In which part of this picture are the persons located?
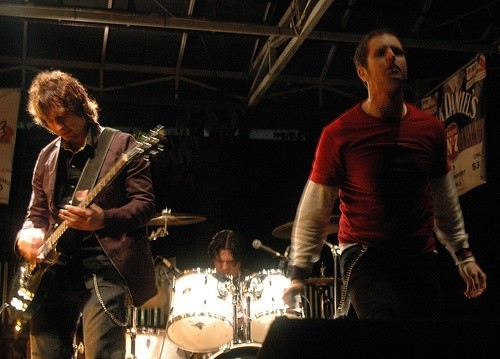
[207,229,250,277]
[282,29,487,321]
[13,71,158,359]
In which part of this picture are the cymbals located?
[303,277,337,289]
[143,213,207,226]
[271,215,341,240]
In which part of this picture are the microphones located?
[252,239,285,258]
[160,256,180,277]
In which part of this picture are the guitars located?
[5,123,169,324]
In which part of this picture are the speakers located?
[258,316,500,359]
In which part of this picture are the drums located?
[230,274,251,344]
[123,326,188,359]
[165,266,236,354]
[242,268,304,344]
[203,341,263,359]
